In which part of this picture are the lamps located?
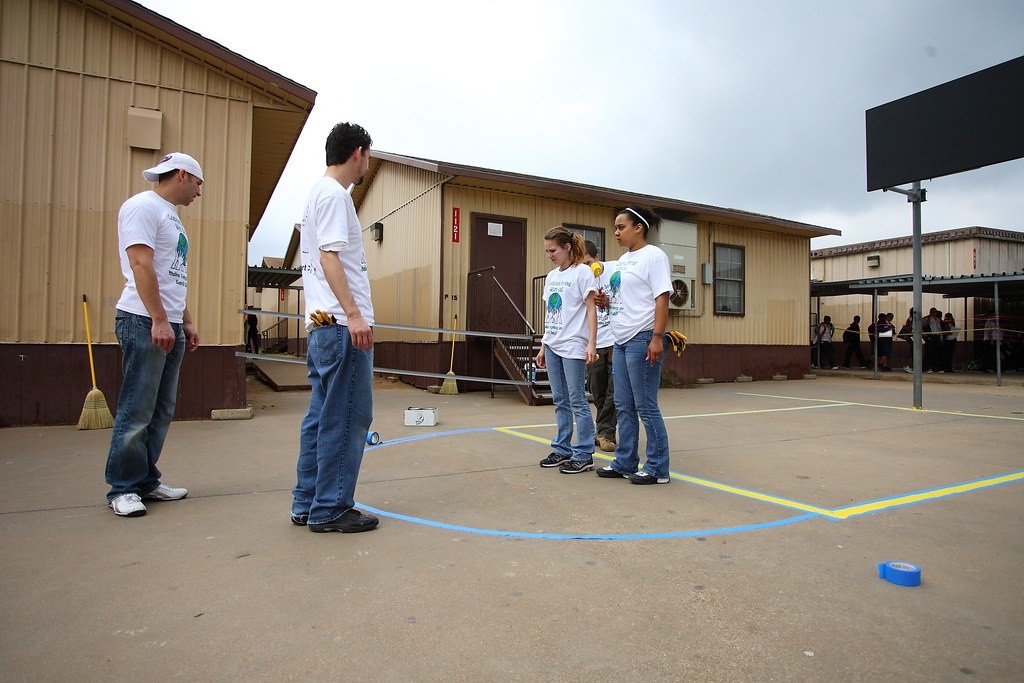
[866,254,880,267]
[370,222,383,241]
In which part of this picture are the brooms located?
[76,293,114,431]
[438,314,459,394]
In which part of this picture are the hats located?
[142,152,204,183]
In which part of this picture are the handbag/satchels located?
[897,317,913,341]
[814,323,825,337]
[946,324,961,340]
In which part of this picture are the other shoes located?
[811,363,821,369]
[884,366,892,372]
[860,365,869,369]
[986,370,993,374]
[936,369,944,374]
[1005,369,1016,372]
[830,365,838,370]
[843,365,851,370]
[925,368,933,374]
[903,365,914,374]
[878,363,884,372]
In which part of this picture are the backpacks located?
[868,322,885,339]
[843,326,859,343]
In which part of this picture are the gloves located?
[309,310,333,328]
[664,331,688,358]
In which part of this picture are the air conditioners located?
[668,274,696,309]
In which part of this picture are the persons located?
[582,240,619,452]
[842,315,868,369]
[903,307,957,374]
[594,205,673,484]
[981,312,1016,373]
[103,151,206,518]
[290,125,381,533]
[535,225,597,475]
[869,312,895,372]
[811,315,840,369]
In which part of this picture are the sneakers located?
[142,482,189,501]
[540,453,572,468]
[109,493,147,517]
[309,509,379,533]
[595,464,631,479]
[596,432,616,452]
[629,470,670,484]
[290,514,308,525]
[559,456,594,474]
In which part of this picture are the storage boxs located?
[403,408,437,428]
